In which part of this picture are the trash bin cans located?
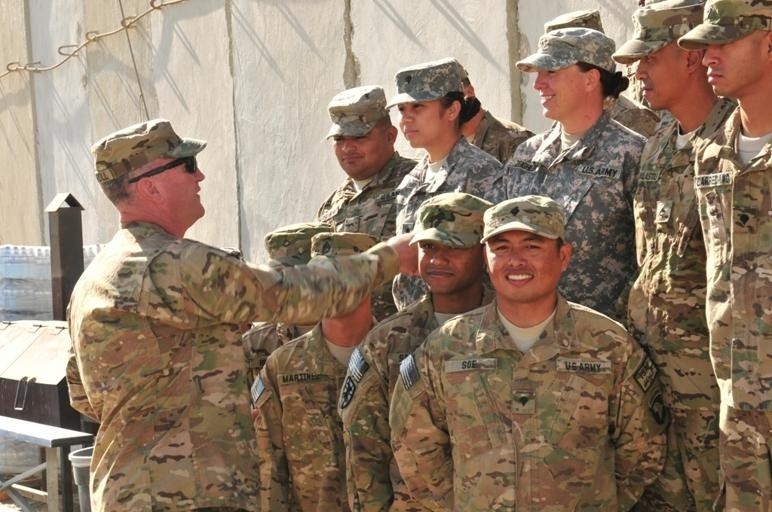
[67,446,94,512]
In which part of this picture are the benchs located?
[0,414,95,511]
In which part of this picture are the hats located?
[263,222,381,267]
[544,8,604,38]
[479,195,566,246]
[384,57,467,111]
[677,1,772,52]
[610,1,706,64]
[91,118,209,184]
[515,27,616,74]
[408,192,495,249]
[326,85,388,141]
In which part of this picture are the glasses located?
[127,156,198,183]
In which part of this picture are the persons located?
[453,57,536,164]
[497,28,650,330]
[545,10,660,139]
[64,119,418,510]
[611,2,726,509]
[338,193,495,510]
[384,57,503,312]
[241,223,334,389]
[250,231,380,510]
[313,85,419,319]
[676,2,771,510]
[390,197,666,510]
